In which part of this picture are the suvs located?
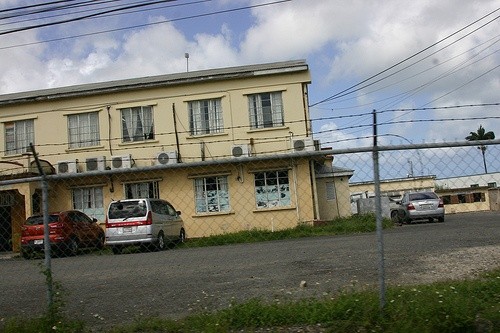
[19,209,105,259]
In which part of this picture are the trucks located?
[350,191,400,216]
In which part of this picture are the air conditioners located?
[84,155,107,172]
[110,154,132,169]
[57,158,80,175]
[313,139,321,151]
[230,143,251,157]
[291,138,315,152]
[154,150,178,166]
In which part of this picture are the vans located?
[104,199,186,255]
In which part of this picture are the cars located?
[396,190,445,223]
